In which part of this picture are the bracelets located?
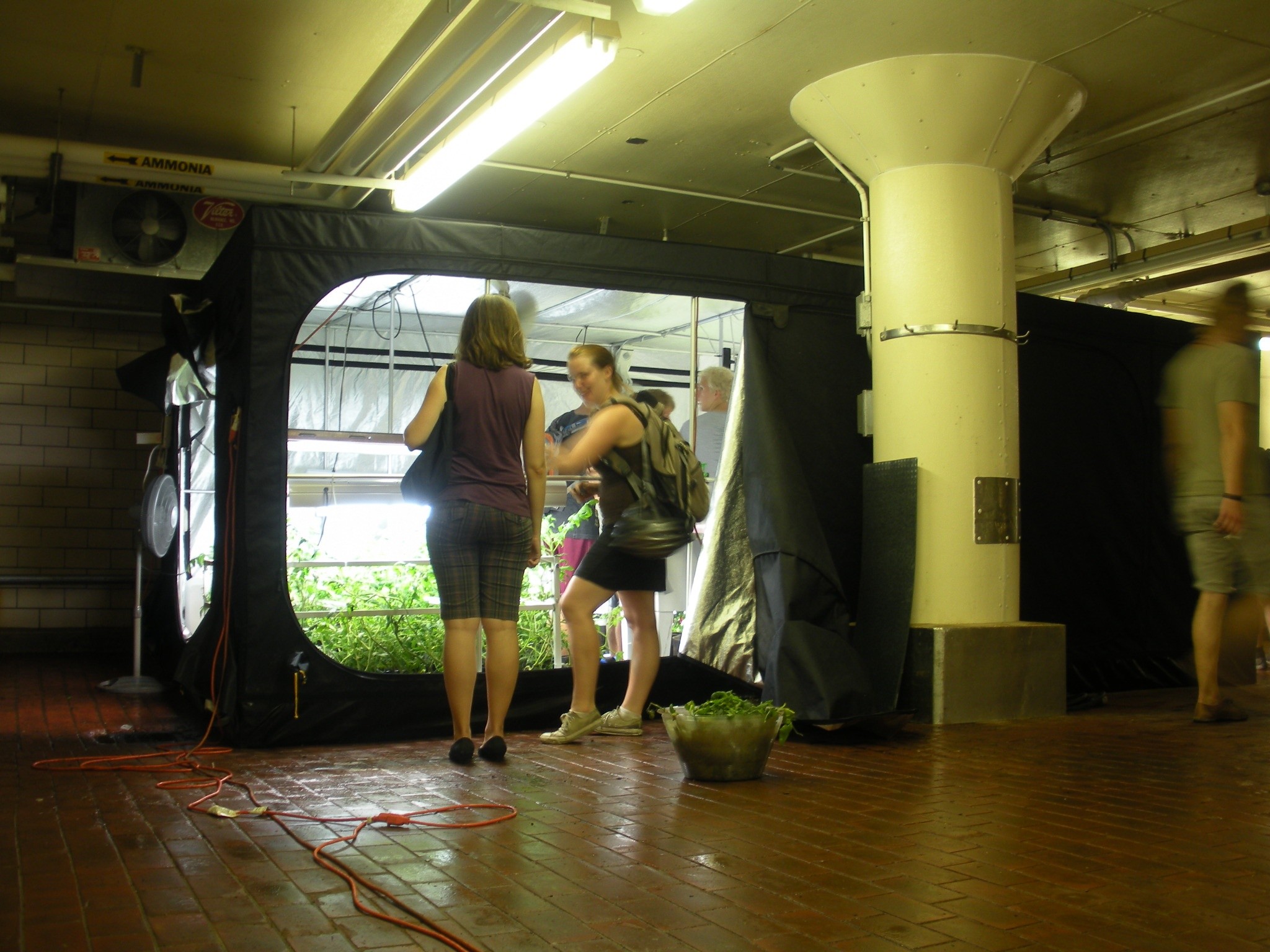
[1221,491,1243,503]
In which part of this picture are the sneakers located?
[540,708,601,744]
[594,705,642,736]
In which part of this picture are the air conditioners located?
[70,178,252,286]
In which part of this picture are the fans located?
[99,471,184,698]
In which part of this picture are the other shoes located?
[478,735,508,761]
[448,737,475,761]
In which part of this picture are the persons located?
[537,344,699,745]
[604,387,677,661]
[396,291,548,765]
[1160,279,1270,721]
[540,391,608,667]
[675,367,737,508]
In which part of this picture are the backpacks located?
[588,398,711,524]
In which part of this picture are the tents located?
[114,200,1263,754]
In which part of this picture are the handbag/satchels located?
[400,400,456,503]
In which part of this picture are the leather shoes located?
[1193,699,1248,723]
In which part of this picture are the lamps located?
[630,0,699,17]
[385,14,622,216]
